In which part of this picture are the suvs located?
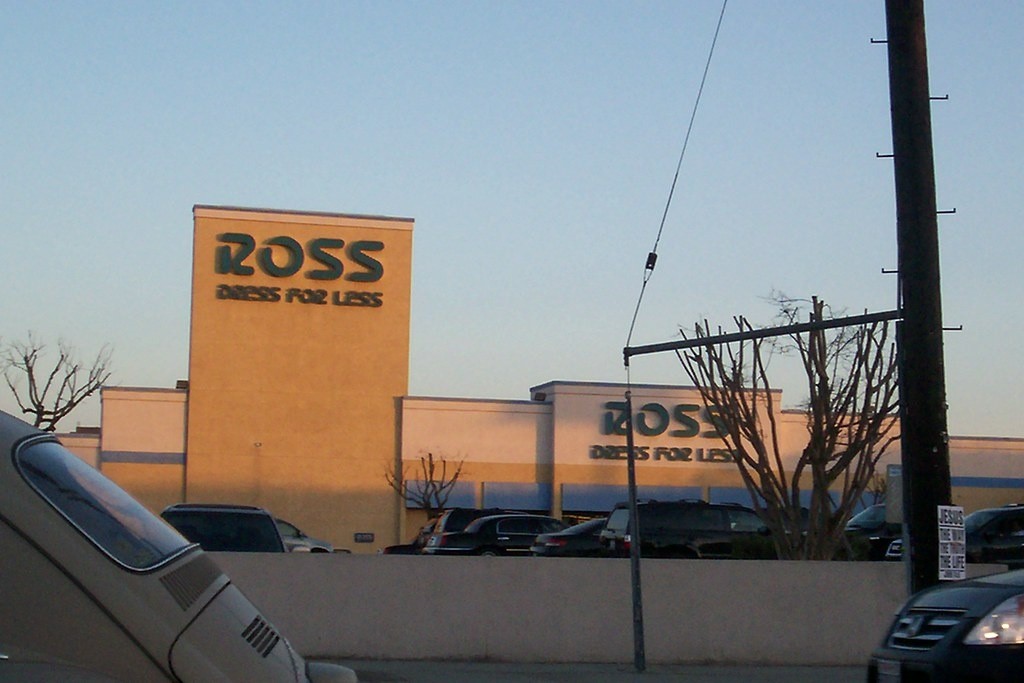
[160,504,351,555]
[381,500,834,561]
[827,500,1024,565]
[0,408,362,683]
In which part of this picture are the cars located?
[868,567,1024,683]
